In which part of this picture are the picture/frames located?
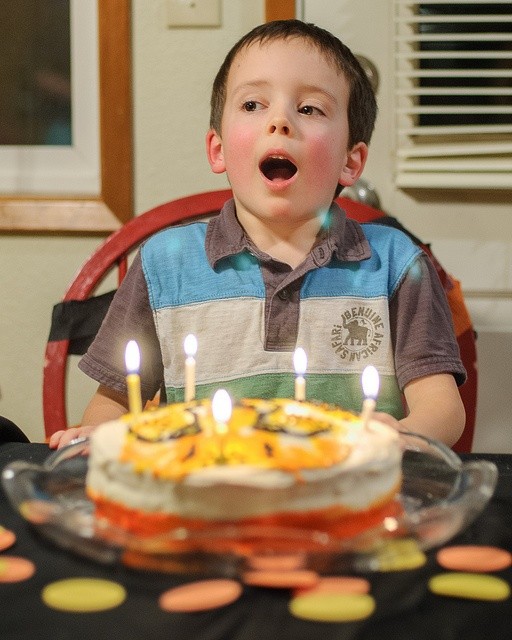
[0,0,135,237]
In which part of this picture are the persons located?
[49,22,467,452]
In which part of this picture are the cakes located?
[87,397,404,564]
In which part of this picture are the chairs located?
[42,187,477,457]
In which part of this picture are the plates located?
[3,429,497,585]
